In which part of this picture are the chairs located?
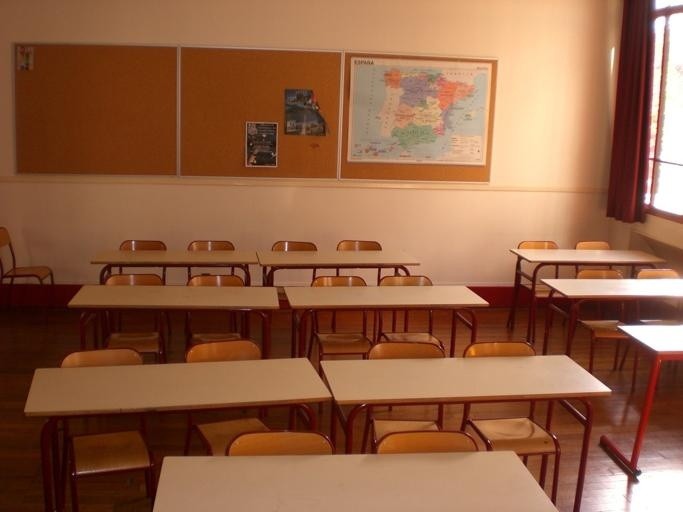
[0,227,58,323]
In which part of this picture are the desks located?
[23,357,333,512]
